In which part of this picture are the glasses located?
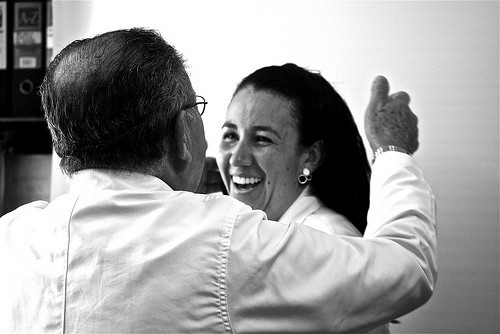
[182,95,208,117]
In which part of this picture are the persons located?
[215,63,390,334]
[0,28,440,334]
